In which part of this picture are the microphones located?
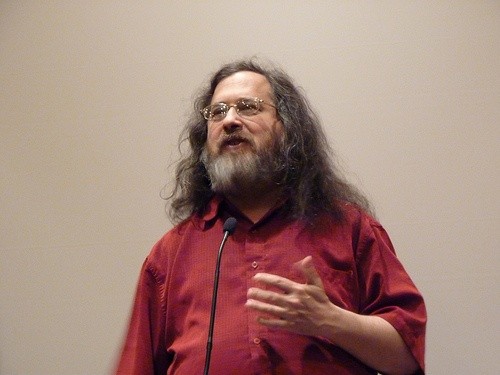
[203,217,237,375]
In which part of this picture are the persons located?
[114,60,428,375]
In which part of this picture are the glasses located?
[200,97,279,121]
